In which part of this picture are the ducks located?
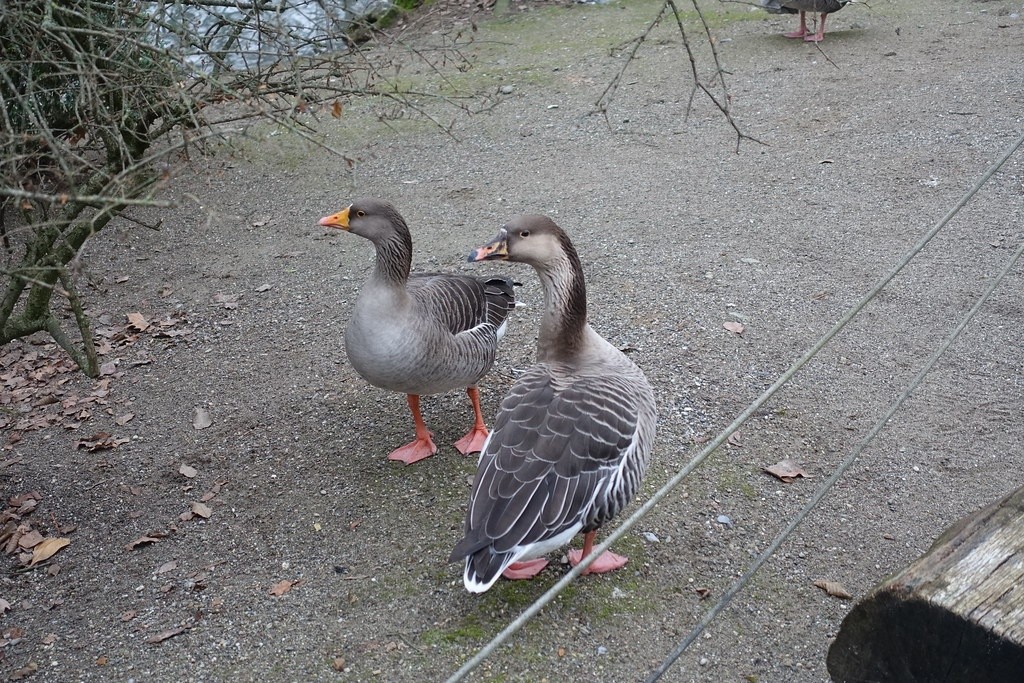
[446,211,657,593]
[760,0,852,43]
[316,196,523,467]
